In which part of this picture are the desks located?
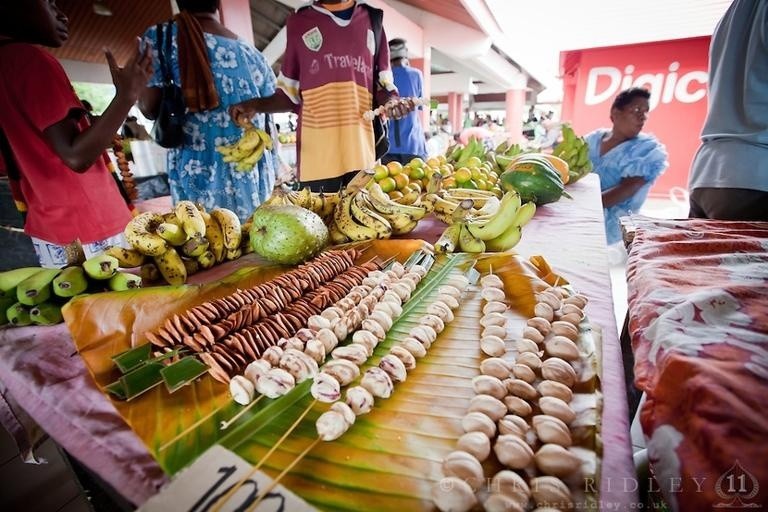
[1,169,639,512]
[616,210,767,511]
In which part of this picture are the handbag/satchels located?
[148,84,187,149]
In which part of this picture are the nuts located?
[145,248,589,512]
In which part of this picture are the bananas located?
[0,125,594,329]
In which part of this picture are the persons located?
[421,103,553,140]
[138,0,277,226]
[0,0,155,269]
[230,0,411,194]
[688,0,767,221]
[82,98,152,140]
[380,39,427,166]
[275,111,300,147]
[586,87,669,267]
